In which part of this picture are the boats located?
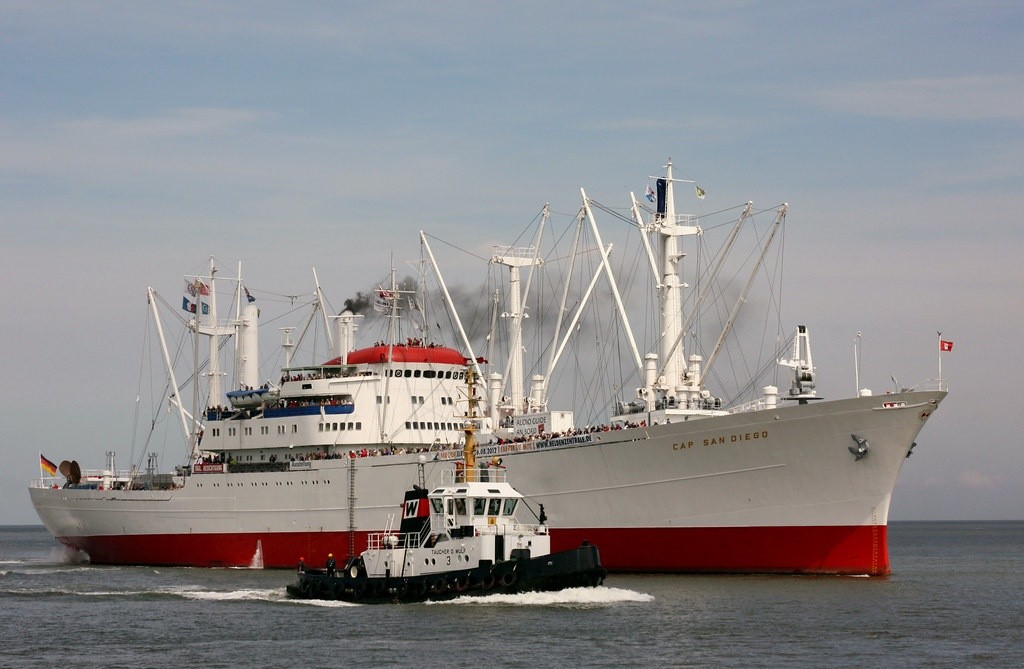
[226,389,281,408]
[286,360,611,606]
[25,164,947,582]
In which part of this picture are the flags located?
[182,277,210,316]
[40,453,58,476]
[941,341,953,352]
[646,184,658,202]
[695,185,708,199]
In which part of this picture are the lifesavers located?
[500,570,519,588]
[373,581,385,594]
[414,581,428,596]
[480,572,496,590]
[398,582,410,596]
[356,584,366,595]
[456,574,470,591]
[396,370,401,376]
[433,578,448,595]
[453,372,457,379]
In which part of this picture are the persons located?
[374,337,442,348]
[488,418,671,446]
[203,440,465,463]
[50,483,183,490]
[206,371,368,414]
